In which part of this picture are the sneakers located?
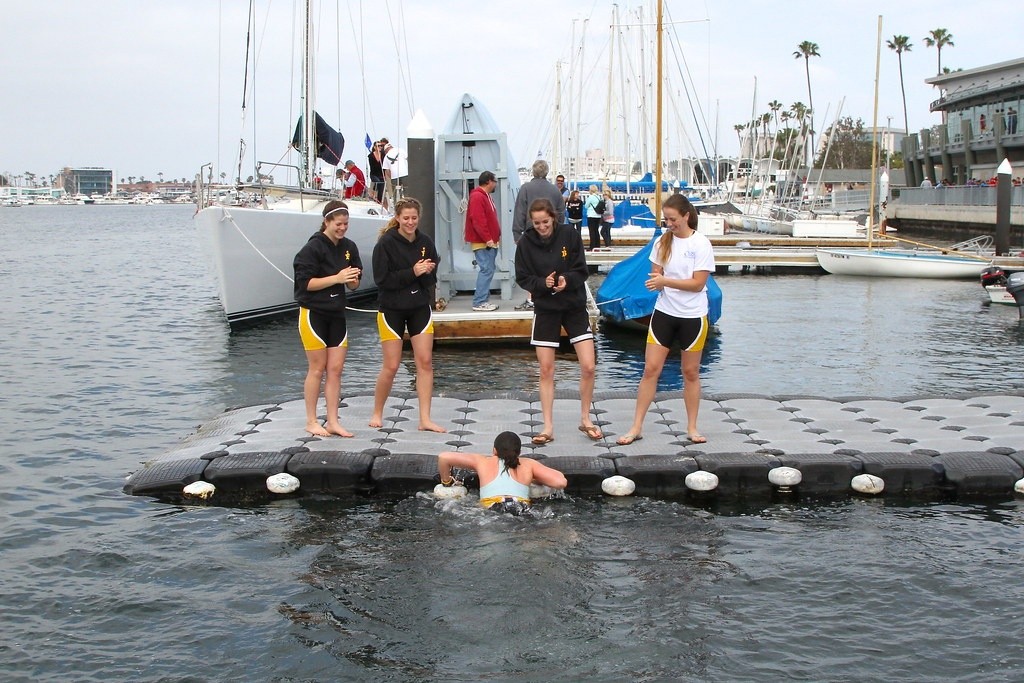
[473,302,499,310]
[515,299,535,311]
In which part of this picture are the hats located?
[336,169,343,179]
[344,160,354,169]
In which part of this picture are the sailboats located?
[190,0,422,329]
[597,0,724,333]
[522,2,864,237]
[816,15,995,278]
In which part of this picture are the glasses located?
[557,179,564,182]
[376,145,383,148]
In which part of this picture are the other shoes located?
[586,248,599,251]
[602,247,612,250]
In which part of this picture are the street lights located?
[886,116,895,174]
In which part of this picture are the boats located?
[980,268,1023,304]
[0,190,269,206]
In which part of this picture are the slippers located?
[578,426,603,438]
[616,435,642,445]
[531,434,555,444]
[685,435,706,443]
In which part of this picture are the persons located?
[336,160,365,200]
[437,432,567,512]
[513,160,565,311]
[567,191,582,235]
[616,195,717,444]
[368,198,446,432]
[980,115,989,139]
[515,199,604,444]
[967,176,1024,188]
[368,138,409,207]
[996,107,1017,134]
[293,201,362,437]
[585,184,600,252]
[920,176,952,189]
[464,171,502,311]
[600,190,615,247]
[555,176,569,224]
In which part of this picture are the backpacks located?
[590,195,606,214]
